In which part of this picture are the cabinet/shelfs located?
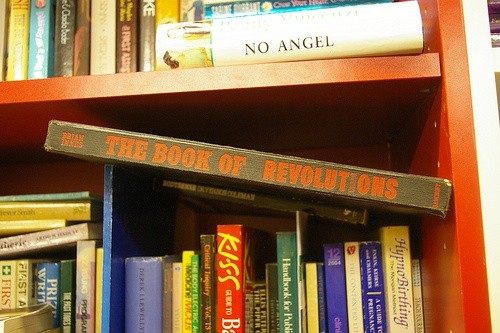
[465,0,500,333]
[0,0,493,333]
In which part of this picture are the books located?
[43,119,452,217]
[161,181,368,226]
[0,191,426,333]
[0,0,425,82]
